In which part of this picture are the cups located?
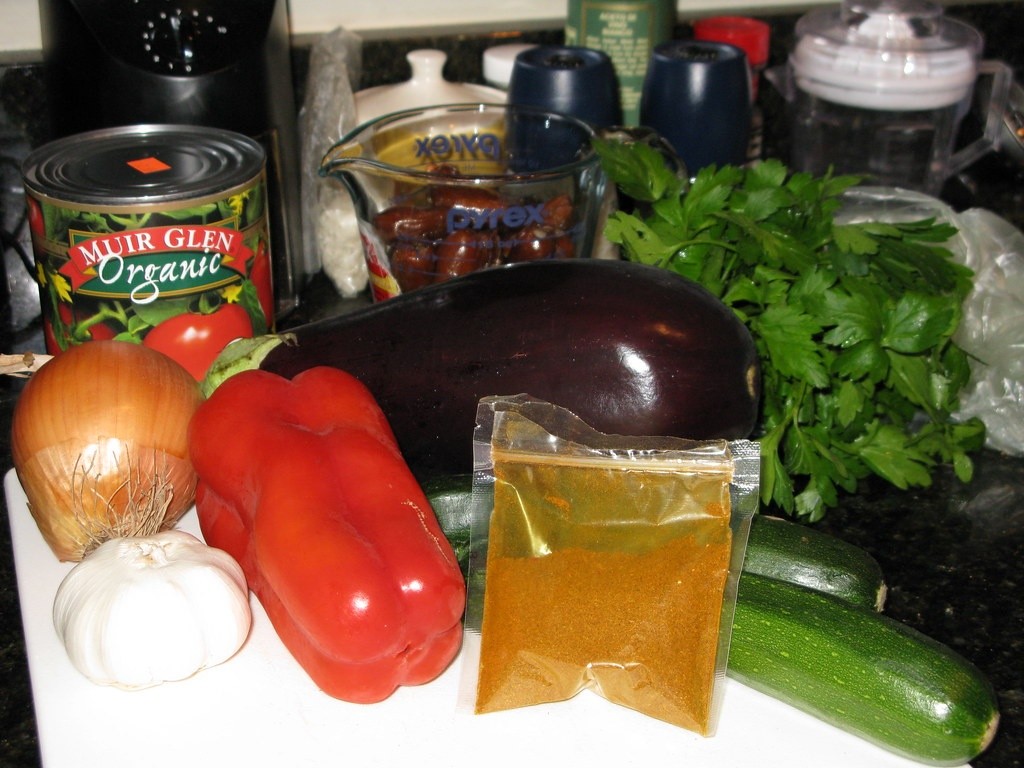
[353,49,507,129]
[640,40,753,179]
[504,46,624,132]
[110,56,241,132]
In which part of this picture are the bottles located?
[482,43,537,92]
[694,16,771,168]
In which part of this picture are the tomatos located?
[46,243,268,385]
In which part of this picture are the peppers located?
[185,366,469,703]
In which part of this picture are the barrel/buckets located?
[19,120,278,360]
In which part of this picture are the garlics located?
[52,529,252,689]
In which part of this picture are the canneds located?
[20,123,270,382]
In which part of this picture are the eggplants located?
[199,255,765,483]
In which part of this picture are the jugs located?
[319,102,688,304]
[764,0,1012,199]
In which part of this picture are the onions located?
[10,340,198,563]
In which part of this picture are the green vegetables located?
[591,131,987,519]
